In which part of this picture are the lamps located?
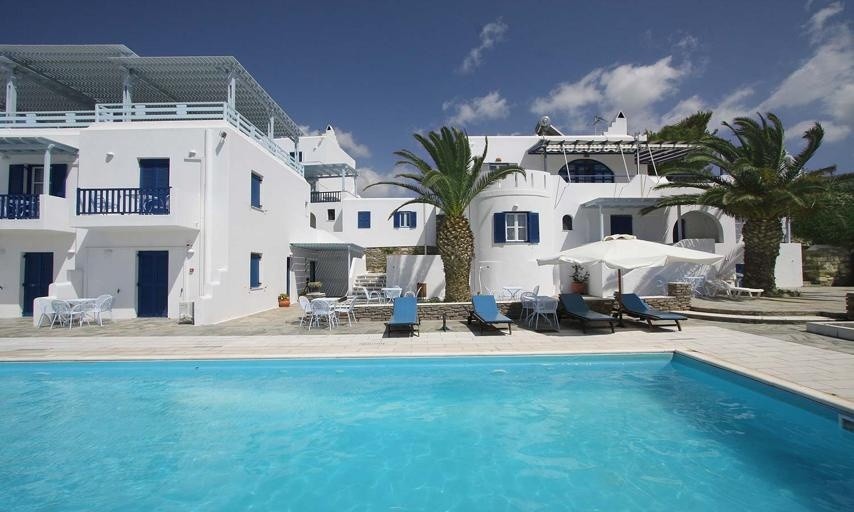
[219,130,227,143]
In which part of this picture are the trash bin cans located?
[417,282,427,303]
[736,264,745,289]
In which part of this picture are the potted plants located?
[277,293,290,307]
[307,281,321,292]
[567,264,590,294]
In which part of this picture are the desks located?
[380,286,402,304]
[305,292,325,298]
[309,297,341,328]
[59,298,99,327]
[525,296,554,330]
[502,287,523,301]
[128,193,158,215]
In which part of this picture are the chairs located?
[335,295,357,327]
[468,294,513,335]
[306,299,338,331]
[534,295,561,331]
[614,293,688,331]
[298,295,319,328]
[362,287,381,304]
[36,298,56,328]
[382,295,420,340]
[705,278,765,298]
[85,295,113,326]
[50,299,83,330]
[557,293,618,334]
[519,285,539,322]
[89,192,116,212]
[403,286,422,297]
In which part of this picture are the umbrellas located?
[535,234,723,327]
